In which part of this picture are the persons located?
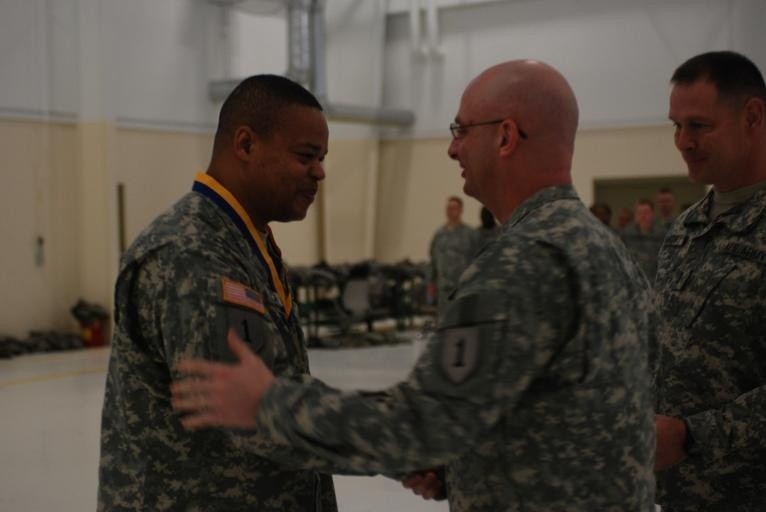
[426,194,473,327]
[94,72,342,512]
[469,203,501,252]
[163,57,661,511]
[588,186,696,289]
[641,44,766,512]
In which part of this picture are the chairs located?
[285,258,437,348]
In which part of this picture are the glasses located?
[450,119,528,141]
